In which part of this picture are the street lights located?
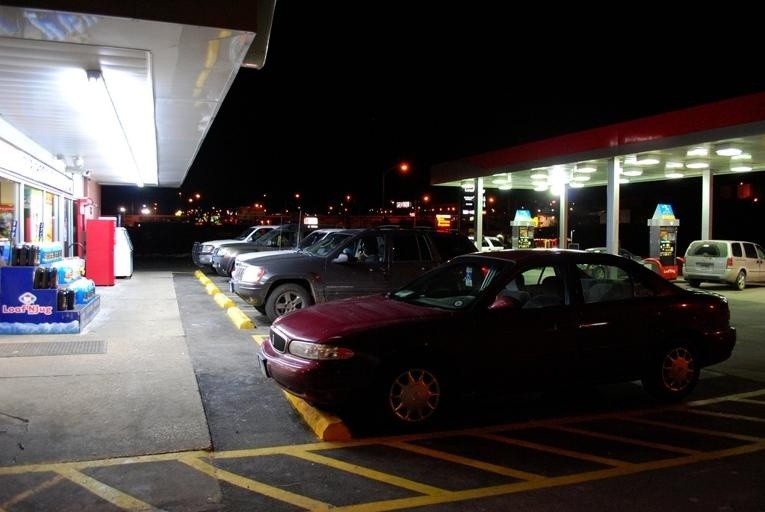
[295,192,300,213]
[413,191,431,227]
[187,191,201,224]
[345,196,351,217]
[485,196,495,234]
[381,162,412,227]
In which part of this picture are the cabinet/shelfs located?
[0,262,102,336]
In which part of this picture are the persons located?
[330,238,353,255]
[276,233,288,241]
[255,230,265,239]
[363,246,379,263]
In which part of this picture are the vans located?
[680,239,765,291]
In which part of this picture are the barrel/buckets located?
[11,241,96,312]
